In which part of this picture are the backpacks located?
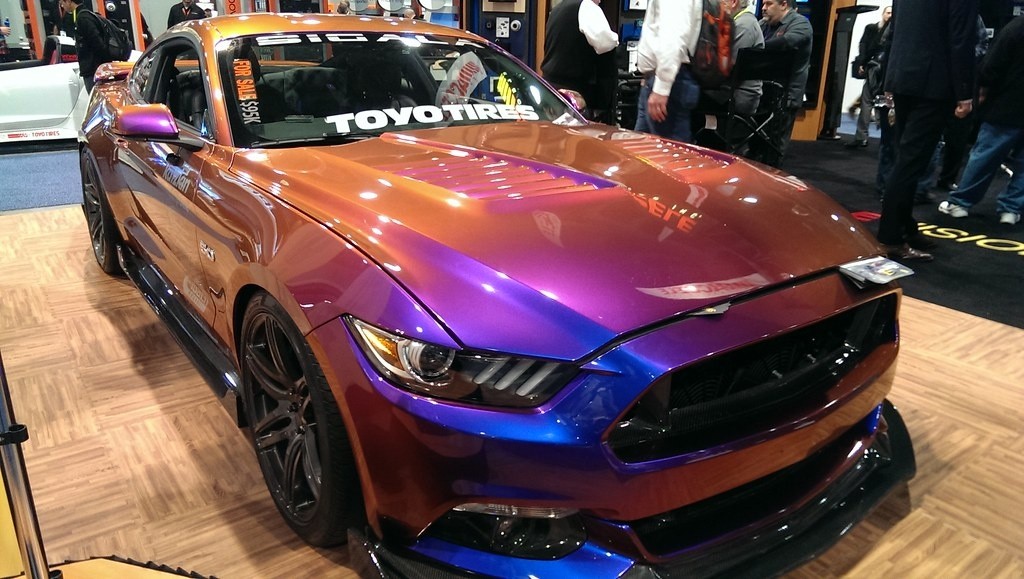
[76,9,132,61]
[688,0,737,90]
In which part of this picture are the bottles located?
[874,94,894,108]
[3,17,10,38]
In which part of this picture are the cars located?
[75,13,920,578]
[0,36,146,143]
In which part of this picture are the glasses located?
[60,0,64,7]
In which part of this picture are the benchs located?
[169,67,346,126]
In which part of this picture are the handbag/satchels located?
[852,60,868,79]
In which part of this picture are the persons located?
[60,0,113,96]
[331,0,357,55]
[848,0,1024,265]
[0,15,11,63]
[632,0,814,171]
[540,0,620,124]
[167,0,218,60]
[403,9,418,19]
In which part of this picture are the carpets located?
[0,152,84,210]
[779,134,1024,330]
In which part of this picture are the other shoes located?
[938,179,959,191]
[912,189,936,201]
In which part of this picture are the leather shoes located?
[846,139,868,146]
[904,229,937,249]
[880,242,934,263]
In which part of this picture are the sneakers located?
[939,200,968,218]
[1000,212,1021,224]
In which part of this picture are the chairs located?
[347,43,417,113]
[192,43,293,130]
[693,47,798,164]
